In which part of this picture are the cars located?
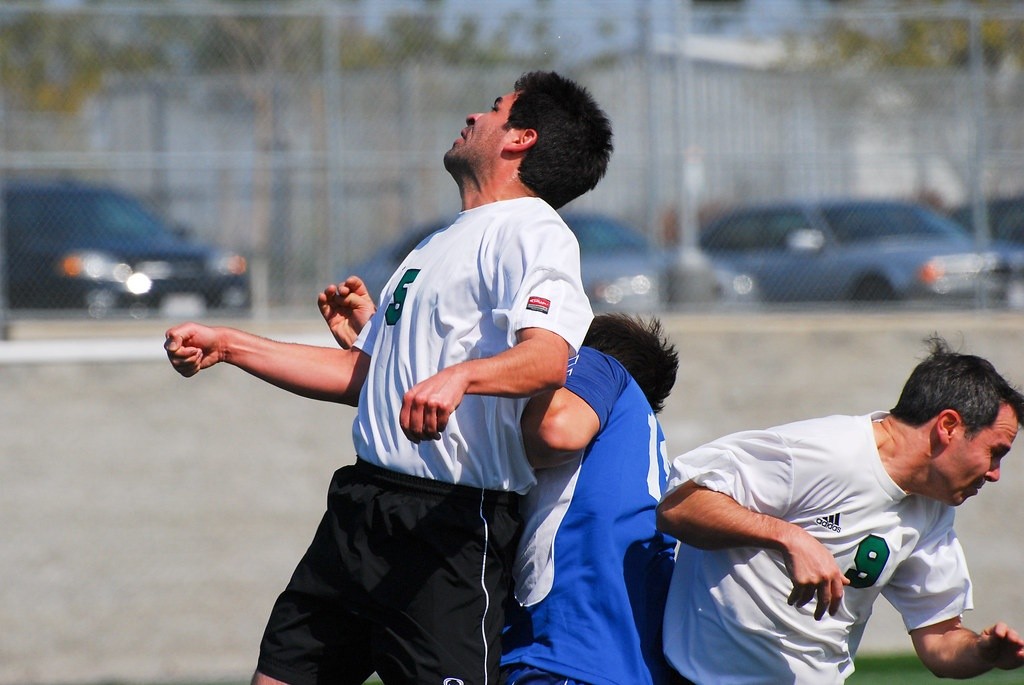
[331,205,754,311]
[1,179,247,313]
[690,197,1024,305]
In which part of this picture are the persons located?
[165,70,613,685]
[313,274,678,685]
[655,334,1023,684]
